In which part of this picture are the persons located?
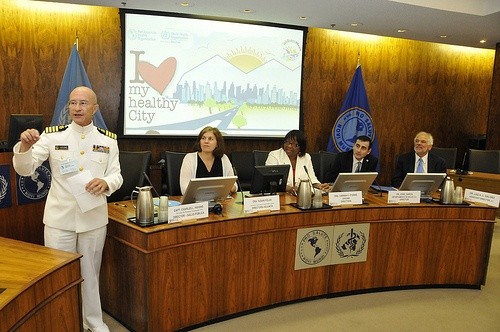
[12,85,123,332]
[180,126,239,195]
[394,132,446,189]
[264,130,331,194]
[336,136,378,185]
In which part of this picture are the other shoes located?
[84,324,110,332]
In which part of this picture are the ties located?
[416,159,423,173]
[356,162,360,171]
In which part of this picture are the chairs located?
[461,148,500,174]
[432,147,457,169]
[104,150,339,203]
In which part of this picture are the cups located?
[313,191,322,208]
[454,187,463,204]
[158,197,168,221]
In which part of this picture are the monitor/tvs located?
[7,114,44,145]
[249,165,290,195]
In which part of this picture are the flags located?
[49,44,107,130]
[328,64,380,172]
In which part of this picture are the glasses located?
[69,100,96,108]
[284,141,298,147]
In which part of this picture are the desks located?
[99,191,499,332]
[0,237,85,332]
[447,169,500,219]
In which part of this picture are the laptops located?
[180,176,238,208]
[331,172,378,201]
[399,173,446,199]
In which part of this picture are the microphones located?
[304,166,315,193]
[456,149,468,174]
[233,167,244,205]
[143,171,160,199]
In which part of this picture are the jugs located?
[440,177,454,204]
[292,180,312,209]
[131,186,154,224]
